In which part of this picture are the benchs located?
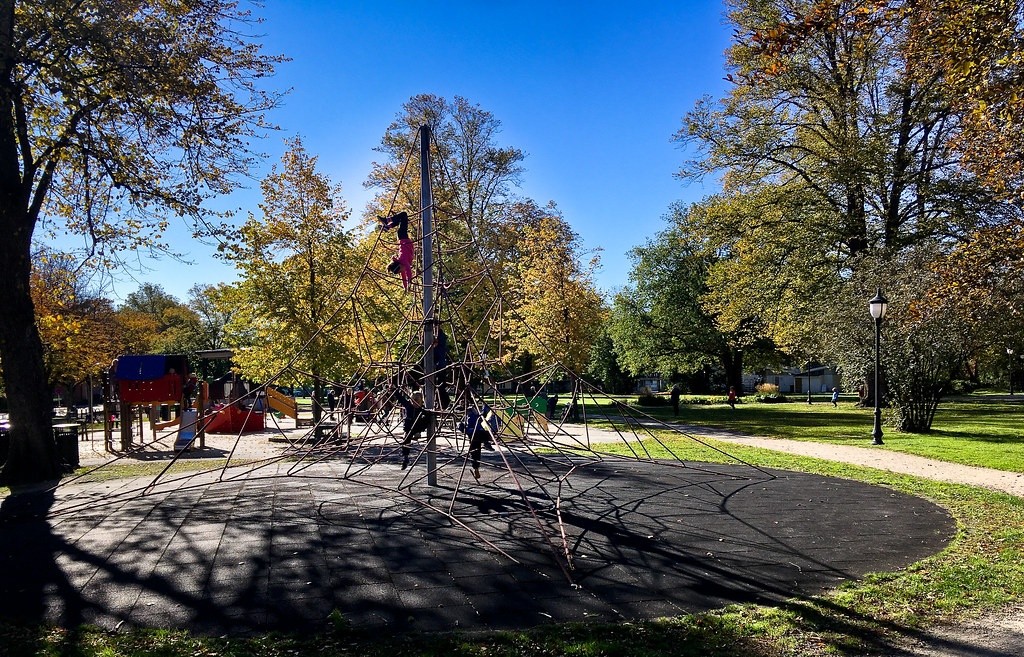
[316,422,337,440]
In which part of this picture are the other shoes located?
[377,216,389,226]
[413,432,421,440]
[474,471,480,478]
[330,418,337,421]
[386,424,392,426]
[401,459,412,470]
[484,445,495,451]
[377,421,381,425]
[377,224,389,232]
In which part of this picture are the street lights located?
[478,349,487,398]
[1007,348,1014,395]
[806,347,813,404]
[868,288,889,445]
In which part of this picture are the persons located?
[831,387,838,408]
[430,326,453,410]
[727,386,735,412]
[389,383,429,470]
[273,385,292,396]
[342,378,413,426]
[465,403,495,480]
[327,390,337,421]
[547,394,559,420]
[377,211,415,295]
[670,384,679,417]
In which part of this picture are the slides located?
[154,394,200,453]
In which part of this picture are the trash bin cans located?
[52,424,81,465]
